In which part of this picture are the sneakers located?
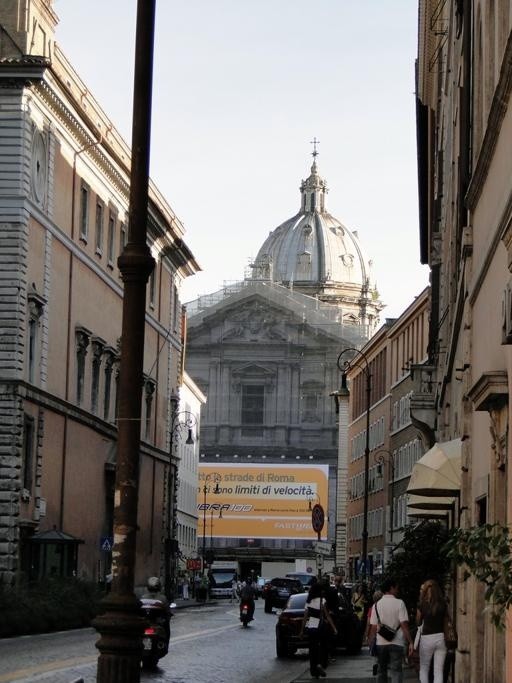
[373,664,378,675]
[312,665,326,679]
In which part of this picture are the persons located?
[298,583,339,678]
[316,575,383,647]
[140,576,171,610]
[237,575,258,597]
[178,570,217,601]
[238,577,257,620]
[415,579,450,682]
[229,575,240,603]
[367,578,414,682]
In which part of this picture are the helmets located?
[246,577,252,584]
[146,577,161,592]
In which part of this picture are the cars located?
[286,572,317,593]
[263,577,306,613]
[274,592,363,659]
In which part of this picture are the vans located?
[207,560,241,600]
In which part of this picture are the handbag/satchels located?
[445,617,456,642]
[378,624,397,642]
[303,616,321,634]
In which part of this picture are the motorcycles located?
[239,596,259,629]
[141,598,177,673]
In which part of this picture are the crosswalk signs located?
[99,535,115,553]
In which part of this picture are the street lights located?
[328,346,376,598]
[165,395,198,603]
[202,473,220,558]
